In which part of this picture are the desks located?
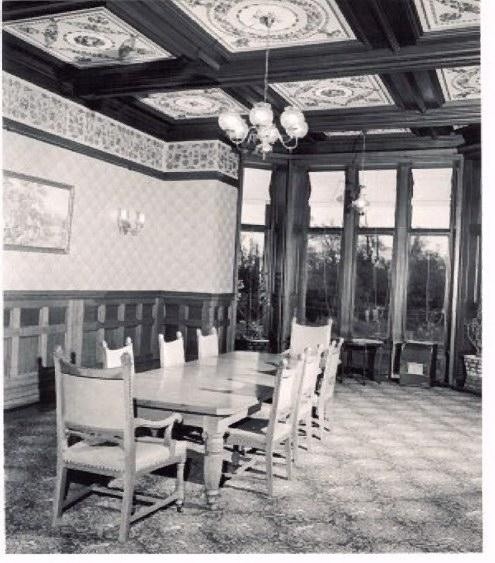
[338,338,384,382]
[130,351,283,507]
[391,339,439,388]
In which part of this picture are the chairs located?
[283,317,332,355]
[199,346,303,500]
[192,326,220,359]
[312,338,344,432]
[158,330,185,370]
[100,335,139,378]
[45,351,187,545]
[232,346,322,453]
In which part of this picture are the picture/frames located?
[2,170,75,256]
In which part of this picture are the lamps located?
[217,17,308,163]
[121,210,145,235]
